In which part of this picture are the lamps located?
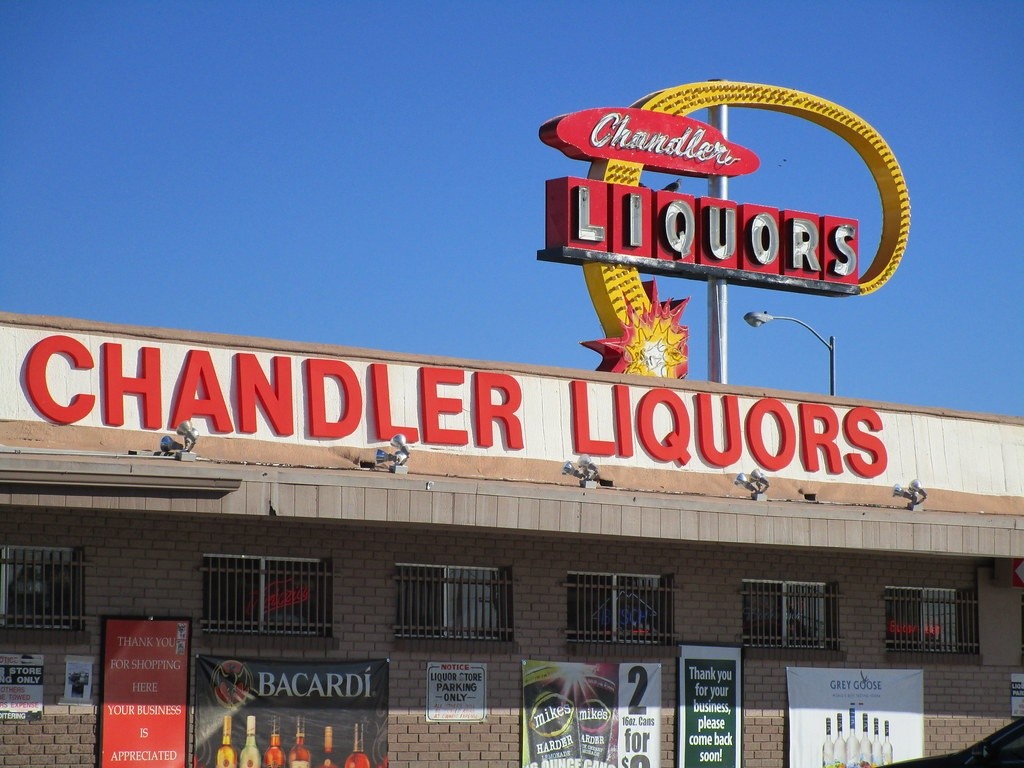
[562,454,600,488]
[735,469,771,500]
[891,479,929,510]
[160,420,200,452]
[374,434,411,466]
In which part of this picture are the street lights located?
[744,311,836,396]
